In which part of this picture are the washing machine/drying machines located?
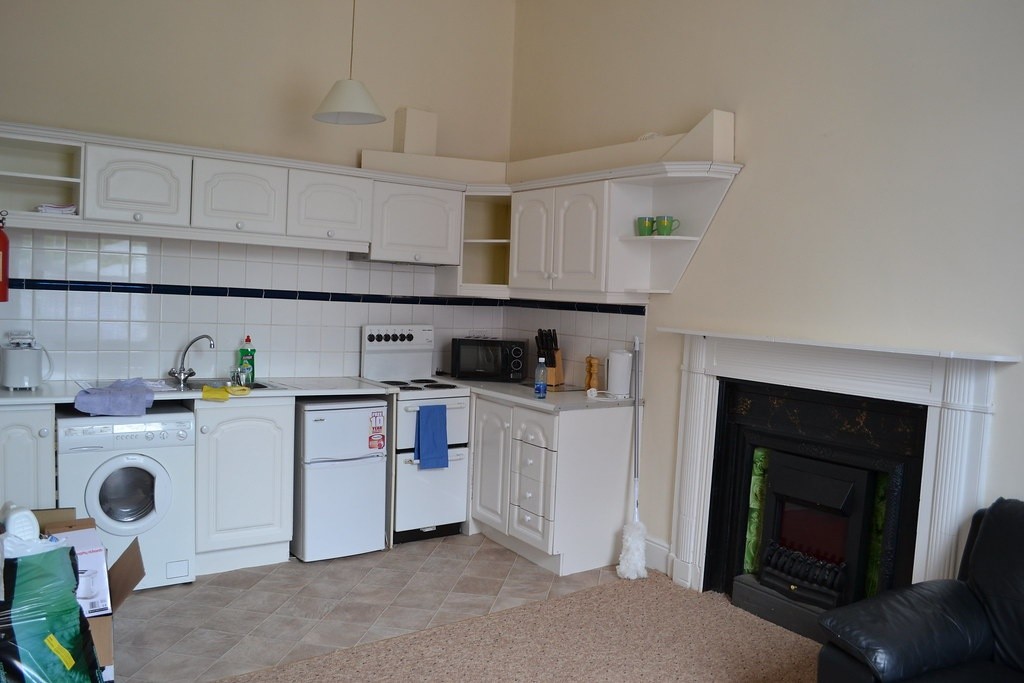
[54,402,197,592]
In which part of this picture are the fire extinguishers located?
[0,217,9,302]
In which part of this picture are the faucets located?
[167,334,215,391]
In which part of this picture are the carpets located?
[212,562,822,683]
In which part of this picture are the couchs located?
[815,496,1024,683]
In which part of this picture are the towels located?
[414,404,449,470]
[74,376,155,417]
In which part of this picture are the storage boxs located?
[32,505,145,680]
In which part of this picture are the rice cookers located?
[0,334,45,391]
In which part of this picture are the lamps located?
[309,0,388,126]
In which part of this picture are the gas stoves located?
[361,324,471,400]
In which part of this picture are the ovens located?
[393,397,472,544]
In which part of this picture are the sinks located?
[167,377,232,390]
[225,381,269,389]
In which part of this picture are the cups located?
[638,216,657,236]
[656,216,680,236]
[229,365,252,389]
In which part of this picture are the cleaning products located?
[237,335,257,383]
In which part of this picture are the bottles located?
[534,358,547,399]
[238,335,256,382]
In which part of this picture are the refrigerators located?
[290,399,387,563]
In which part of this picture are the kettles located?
[604,348,633,399]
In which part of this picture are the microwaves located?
[451,334,530,384]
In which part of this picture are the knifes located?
[535,328,558,367]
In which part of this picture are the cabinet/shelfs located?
[435,160,744,304]
[1,122,468,267]
[470,388,645,577]
[0,403,55,508]
[193,395,293,579]
